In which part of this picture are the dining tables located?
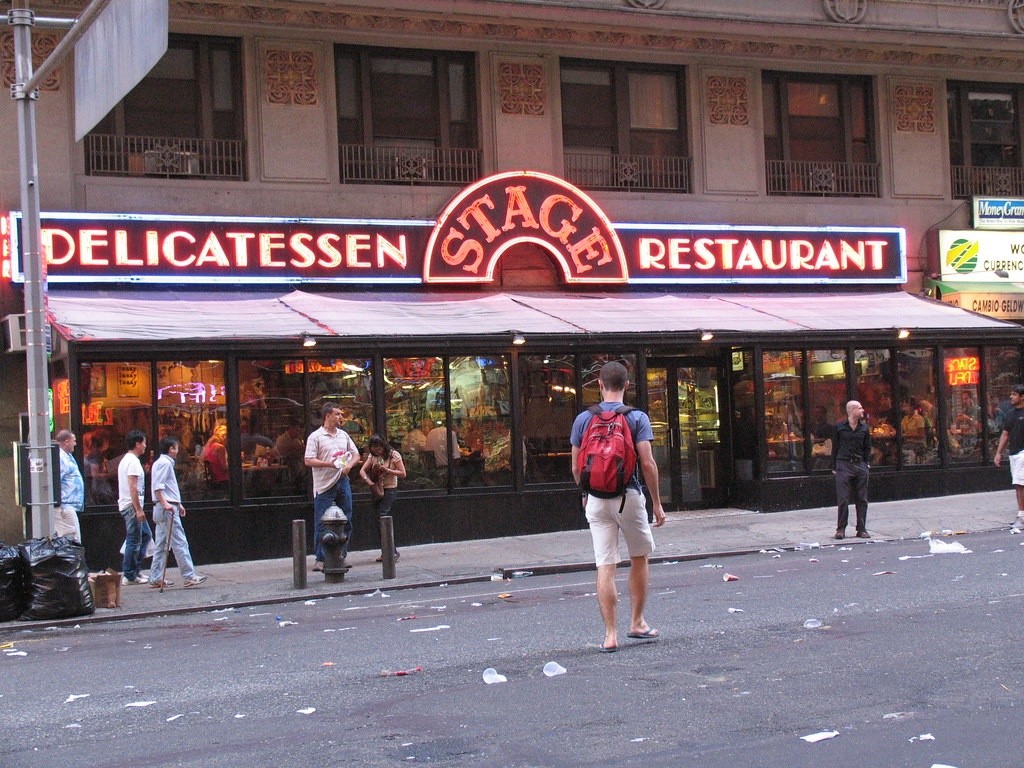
[241,462,287,497]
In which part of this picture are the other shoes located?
[150,580,174,587]
[121,573,149,585]
[395,552,400,561]
[344,559,352,567]
[857,531,870,538]
[836,527,845,538]
[376,557,382,562]
[1009,518,1024,529]
[184,575,208,587]
[312,561,324,570]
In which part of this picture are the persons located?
[831,401,871,539]
[150,436,208,588]
[994,385,1024,530]
[360,435,406,562]
[240,358,375,435]
[53,429,84,544]
[791,388,1014,466]
[83,410,306,505]
[304,402,361,571]
[570,361,666,651]
[401,411,527,487]
[118,430,152,586]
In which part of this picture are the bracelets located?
[363,476,369,481]
[387,469,390,474]
[868,465,871,468]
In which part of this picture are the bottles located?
[150,451,154,468]
[969,417,973,429]
[381,459,384,465]
[873,428,878,438]
[241,452,245,467]
[266,447,270,467]
[257,458,262,467]
[882,421,889,437]
[783,424,788,440]
[790,431,794,440]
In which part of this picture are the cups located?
[91,464,99,474]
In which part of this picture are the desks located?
[528,451,573,483]
[768,436,826,471]
[869,433,913,467]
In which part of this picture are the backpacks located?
[577,405,638,500]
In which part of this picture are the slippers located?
[599,643,617,651]
[627,626,659,638]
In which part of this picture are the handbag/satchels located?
[370,464,384,499]
[120,536,155,558]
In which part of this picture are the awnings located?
[44,292,1024,341]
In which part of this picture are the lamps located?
[303,336,316,346]
[701,332,713,340]
[898,328,909,338]
[513,335,525,345]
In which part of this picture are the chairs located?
[417,450,447,487]
[204,460,229,500]
